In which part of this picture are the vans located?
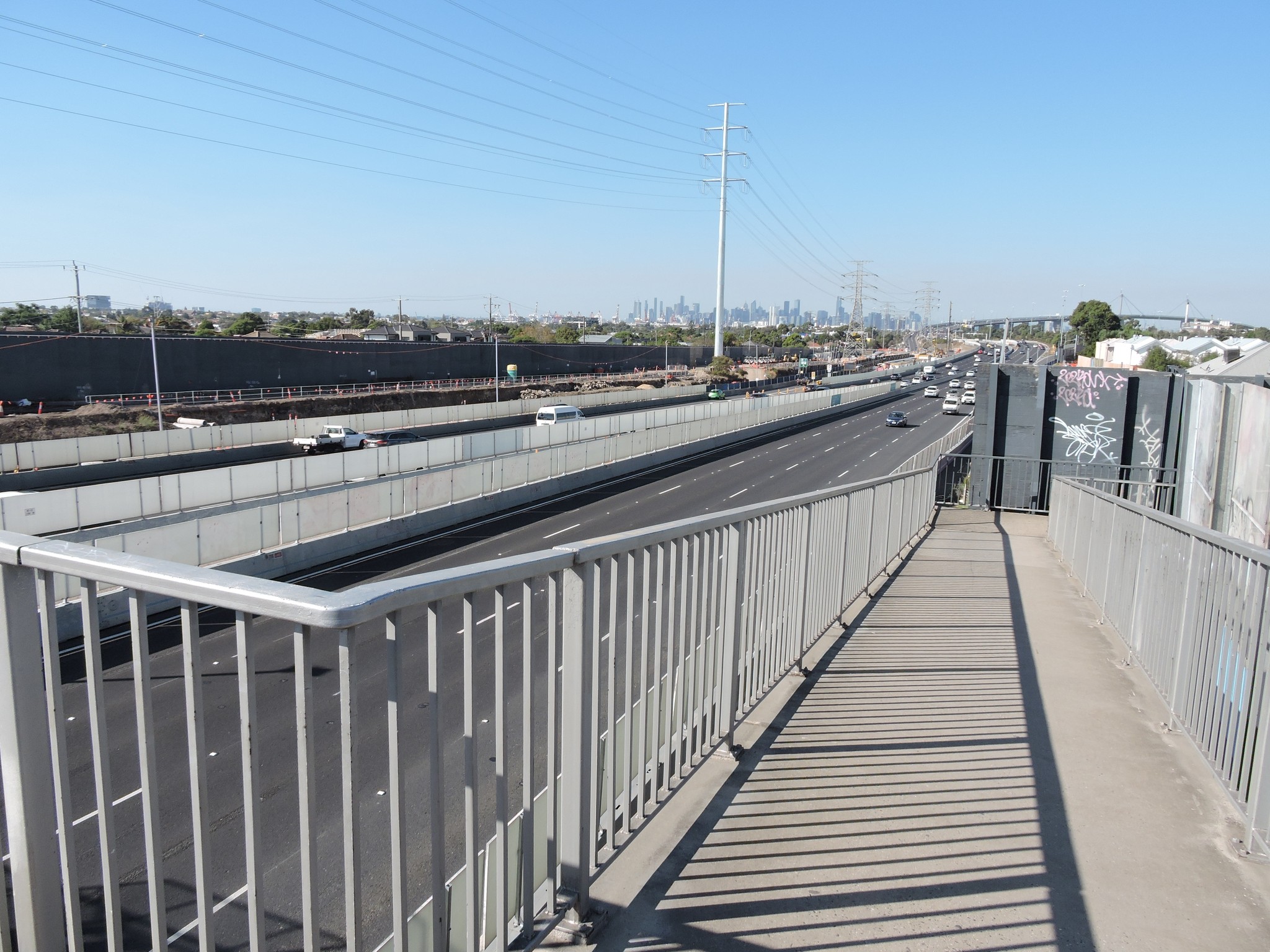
[535,405,586,426]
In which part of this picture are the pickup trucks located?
[292,425,368,455]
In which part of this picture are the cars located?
[708,389,726,400]
[973,339,1044,367]
[749,393,770,399]
[890,362,976,415]
[885,411,908,428]
[870,378,881,384]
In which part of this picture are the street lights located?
[705,332,713,347]
[824,339,841,359]
[656,327,667,345]
[798,351,803,374]
[872,308,915,352]
[773,337,778,347]
[749,334,755,350]
[756,344,758,378]
[665,340,670,388]
[494,333,498,403]
[960,284,1086,346]
[1156,310,1163,334]
[627,337,630,345]
[812,339,814,357]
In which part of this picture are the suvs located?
[543,403,569,407]
[362,430,430,450]
[797,376,830,392]
